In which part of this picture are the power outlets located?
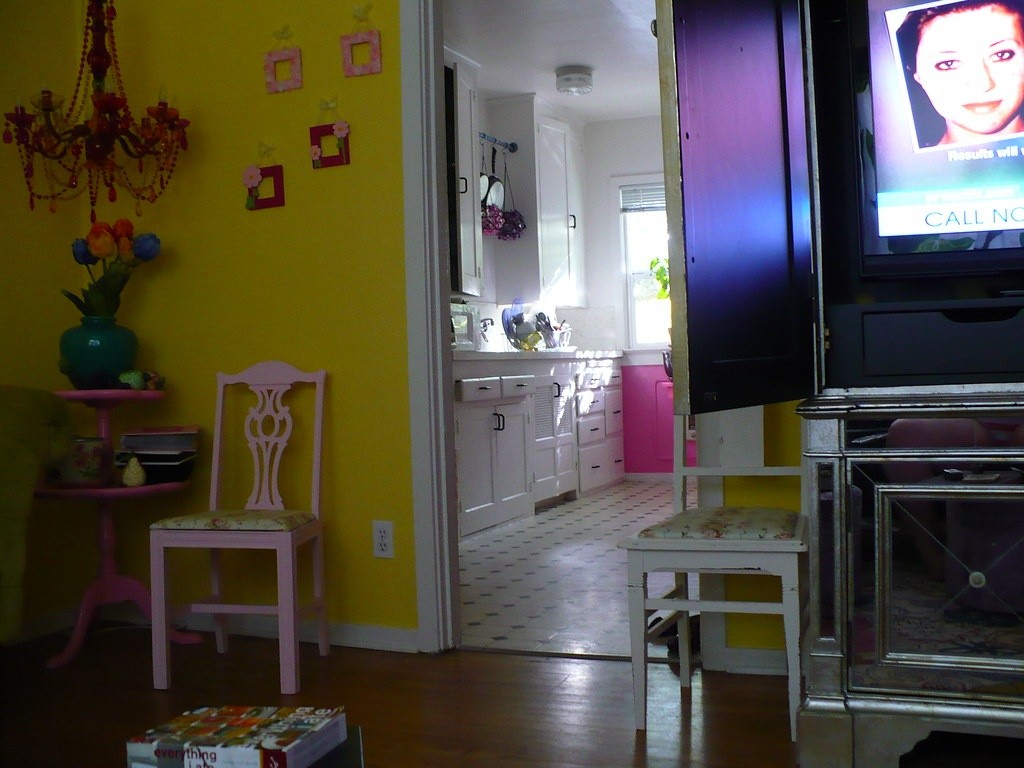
[373,521,393,560]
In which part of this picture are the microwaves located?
[450,303,481,351]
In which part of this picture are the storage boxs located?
[124,707,365,768]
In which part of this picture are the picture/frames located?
[263,47,302,94]
[340,30,383,77]
[241,164,286,210]
[309,121,350,169]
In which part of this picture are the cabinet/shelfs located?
[488,93,588,310]
[451,359,622,537]
[444,46,485,298]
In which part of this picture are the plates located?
[547,346,577,352]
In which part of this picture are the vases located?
[58,319,138,390]
[498,232,524,241]
[483,230,499,235]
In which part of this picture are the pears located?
[120,457,145,487]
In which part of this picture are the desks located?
[32,390,206,668]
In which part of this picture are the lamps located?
[556,66,594,95]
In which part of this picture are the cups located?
[520,331,541,350]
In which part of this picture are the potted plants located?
[649,257,673,377]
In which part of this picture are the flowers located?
[501,210,527,233]
[482,204,503,229]
[59,217,161,315]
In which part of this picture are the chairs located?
[150,361,328,693]
[617,413,814,741]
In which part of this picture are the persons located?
[896,0,1024,146]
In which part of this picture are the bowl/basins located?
[543,331,570,348]
